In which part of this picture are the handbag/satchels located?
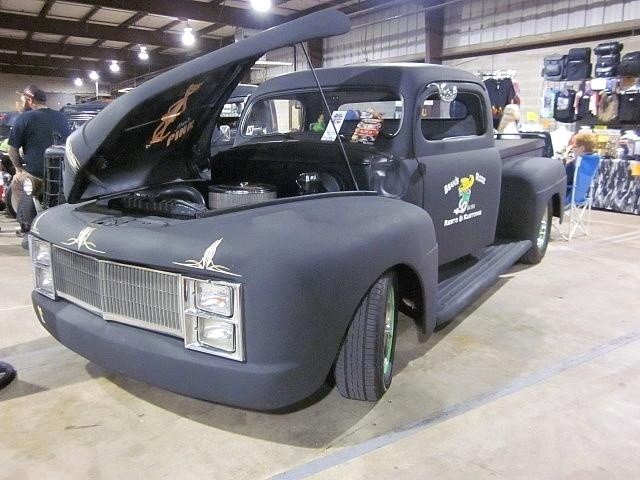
[566,49,590,79]
[620,92,640,124]
[553,90,575,122]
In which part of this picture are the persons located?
[7,83,72,238]
[563,133,596,205]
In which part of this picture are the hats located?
[15,86,47,102]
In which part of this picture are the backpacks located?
[545,54,568,81]
